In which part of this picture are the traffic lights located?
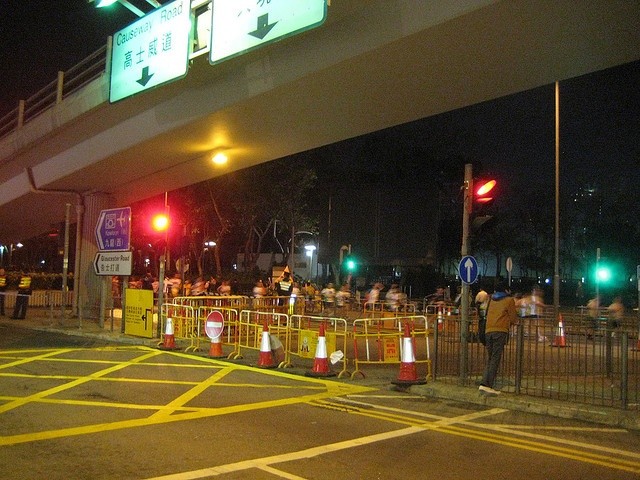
[344,257,360,277]
[593,263,615,286]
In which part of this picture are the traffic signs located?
[109,0,192,104]
[205,311,224,339]
[124,288,154,339]
[209,0,328,66]
[94,206,131,252]
[93,251,133,275]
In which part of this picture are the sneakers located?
[478,385,501,397]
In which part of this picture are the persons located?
[511,291,520,337]
[478,283,518,397]
[335,283,351,308]
[9,268,33,320]
[584,298,600,340]
[319,283,335,313]
[253,280,266,321]
[0,268,8,317]
[432,286,473,315]
[291,282,321,302]
[365,283,384,311]
[386,284,409,312]
[277,271,293,306]
[475,286,489,319]
[518,294,529,337]
[526,288,547,342]
[107,272,231,308]
[265,277,272,300]
[606,296,625,339]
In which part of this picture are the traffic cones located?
[391,323,427,384]
[447,308,450,316]
[209,334,228,358]
[257,320,276,369]
[437,306,443,335]
[305,323,336,377]
[163,309,176,348]
[549,312,570,347]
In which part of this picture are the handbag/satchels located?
[478,295,491,346]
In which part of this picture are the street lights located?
[304,244,316,280]
[459,180,496,241]
[152,214,167,278]
[205,238,216,291]
